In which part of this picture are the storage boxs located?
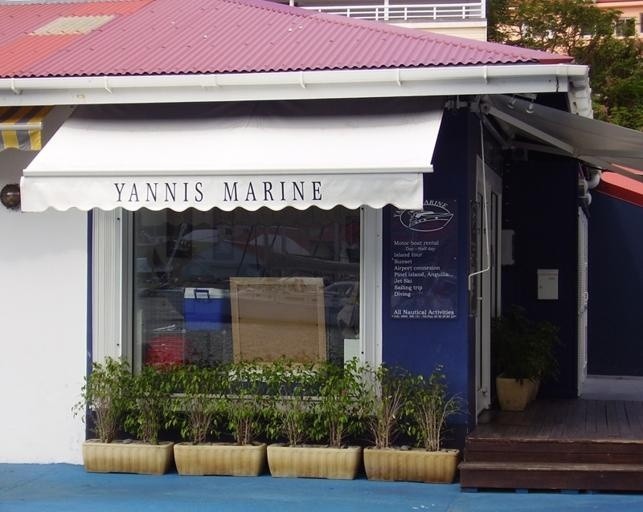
[181,285,231,332]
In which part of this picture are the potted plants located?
[488,304,567,410]
[172,358,266,478]
[70,357,175,478]
[264,357,363,481]
[361,357,467,484]
